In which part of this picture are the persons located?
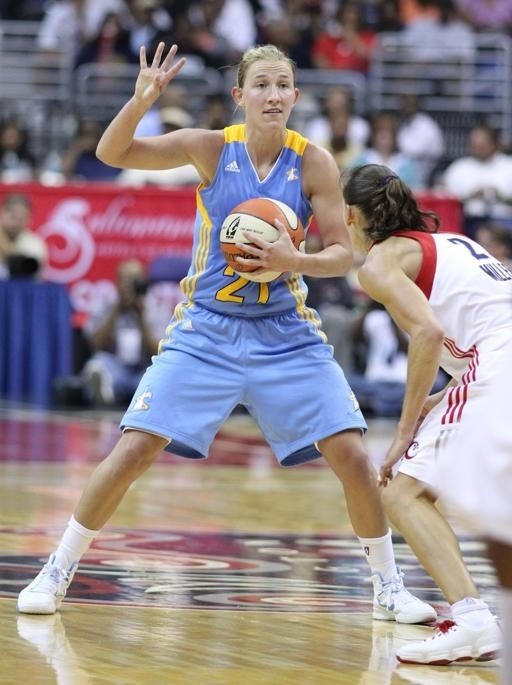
[0,85,510,420]
[35,0,512,129]
[335,159,512,668]
[390,657,511,683]
[13,612,430,685]
[17,38,441,624]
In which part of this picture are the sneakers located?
[18,551,80,614]
[372,569,437,623]
[392,616,505,665]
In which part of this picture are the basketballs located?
[220,198,306,283]
